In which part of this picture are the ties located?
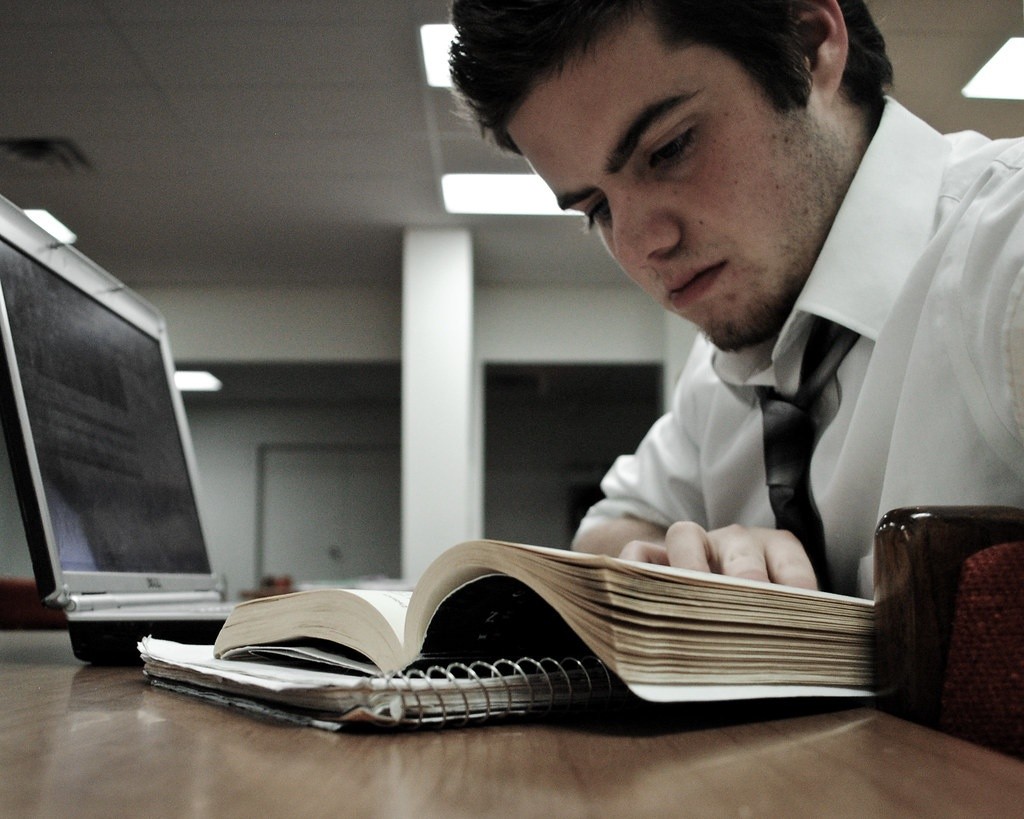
[754,315,862,593]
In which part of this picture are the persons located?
[442,1,1024,591]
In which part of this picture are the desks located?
[0,630,1024,819]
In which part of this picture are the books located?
[140,539,874,733]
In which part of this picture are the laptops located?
[0,197,244,668]
